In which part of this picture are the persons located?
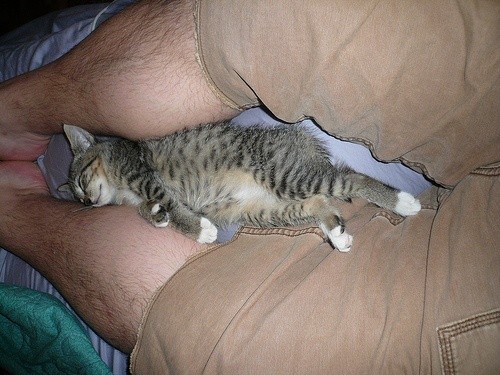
[1,0,500,374]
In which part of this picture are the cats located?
[57,124,421,251]
